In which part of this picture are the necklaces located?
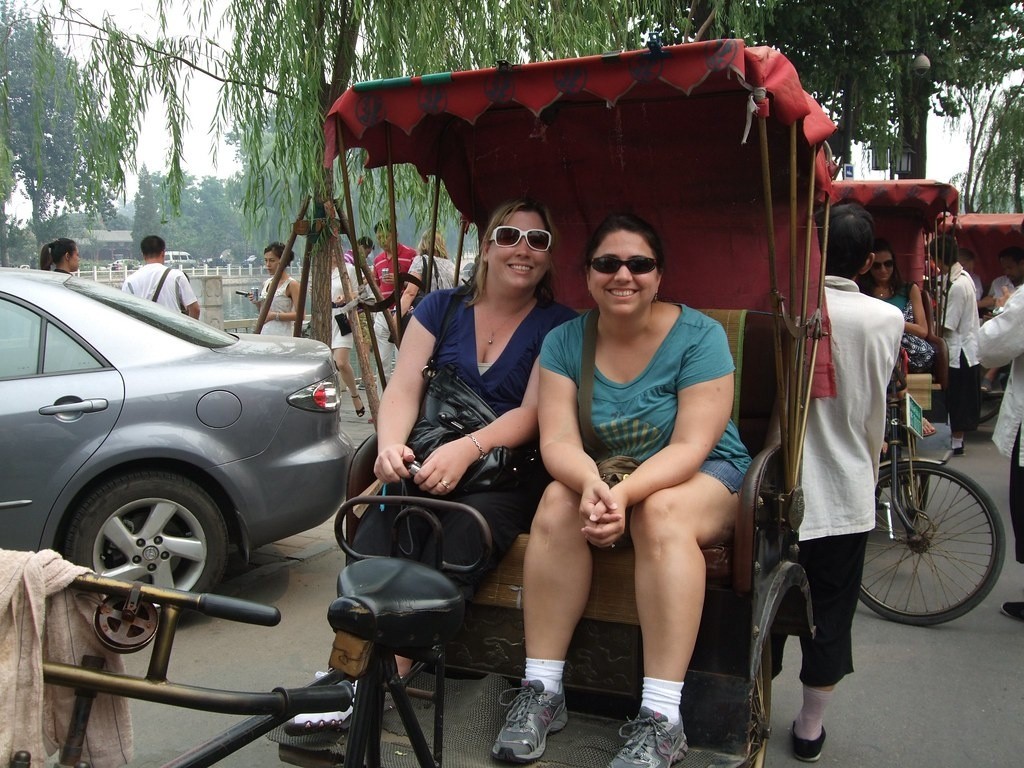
[880,293,884,296]
[478,301,528,345]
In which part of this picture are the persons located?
[856,237,938,457]
[282,196,583,732]
[249,242,305,337]
[475,255,480,264]
[111,261,121,271]
[138,264,143,270]
[331,219,459,425]
[180,270,190,317]
[29,257,37,269]
[121,235,200,321]
[926,203,1024,622]
[41,238,80,276]
[492,213,754,768]
[770,203,906,762]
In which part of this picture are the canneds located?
[250,288,258,301]
[381,268,389,282]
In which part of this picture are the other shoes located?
[791,720,826,763]
[283,668,358,736]
[953,442,965,457]
[358,380,366,389]
[1001,601,1024,621]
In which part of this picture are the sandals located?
[981,377,992,392]
[922,418,936,436]
[352,394,365,417]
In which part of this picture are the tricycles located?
[928,213,1024,426]
[0,38,826,767]
[812,181,1005,628]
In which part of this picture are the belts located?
[332,302,346,308]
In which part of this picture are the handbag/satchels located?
[597,455,643,547]
[388,253,440,343]
[400,364,533,512]
[900,281,937,373]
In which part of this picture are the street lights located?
[840,48,931,180]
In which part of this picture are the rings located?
[610,543,616,549]
[440,479,450,489]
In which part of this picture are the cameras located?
[407,461,421,474]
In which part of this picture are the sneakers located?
[491,680,568,764]
[606,706,689,768]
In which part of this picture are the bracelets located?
[276,312,279,321]
[465,432,486,462]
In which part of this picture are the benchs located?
[414,308,791,696]
[905,292,951,423]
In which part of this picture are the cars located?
[107,259,140,267]
[0,267,354,629]
[203,257,226,268]
[242,257,263,269]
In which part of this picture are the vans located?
[164,251,197,269]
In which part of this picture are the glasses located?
[589,256,658,274]
[489,226,552,251]
[872,260,893,270]
